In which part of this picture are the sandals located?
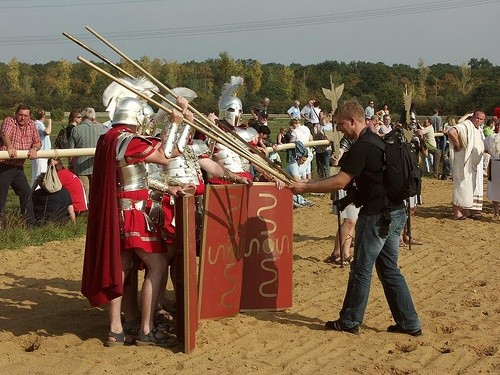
[123,319,141,333]
[136,326,179,347]
[153,305,174,325]
[105,328,137,347]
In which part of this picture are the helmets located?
[137,104,156,137]
[218,96,242,126]
[111,99,146,126]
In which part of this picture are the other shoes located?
[293,202,303,209]
[399,240,407,247]
[387,323,422,336]
[302,201,316,207]
[323,252,354,267]
[467,213,481,220]
[403,234,423,245]
[325,318,359,334]
[459,215,467,220]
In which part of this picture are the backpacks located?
[55,124,68,149]
[357,130,423,204]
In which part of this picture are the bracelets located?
[32,147,37,150]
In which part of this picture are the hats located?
[261,110,268,117]
[81,107,96,119]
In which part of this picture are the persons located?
[290,103,422,336]
[0,75,500,350]
